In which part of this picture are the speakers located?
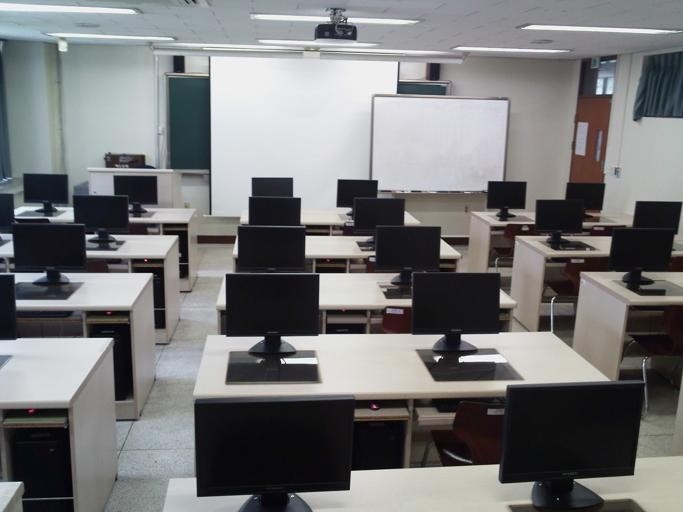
[426,62,440,80]
[172,54,186,73]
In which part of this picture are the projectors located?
[315,24,358,45]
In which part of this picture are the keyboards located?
[431,397,505,412]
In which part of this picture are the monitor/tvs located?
[337,178,441,284]
[412,272,501,356]
[536,182,605,244]
[0,173,160,285]
[0,274,17,340]
[609,201,683,286]
[497,379,645,511]
[487,181,527,217]
[193,395,357,512]
[225,273,319,357]
[238,176,307,273]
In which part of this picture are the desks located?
[88,168,183,207]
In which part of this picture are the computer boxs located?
[9,428,74,512]
[91,324,129,401]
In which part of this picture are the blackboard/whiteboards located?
[166,75,450,171]
[371,95,510,196]
